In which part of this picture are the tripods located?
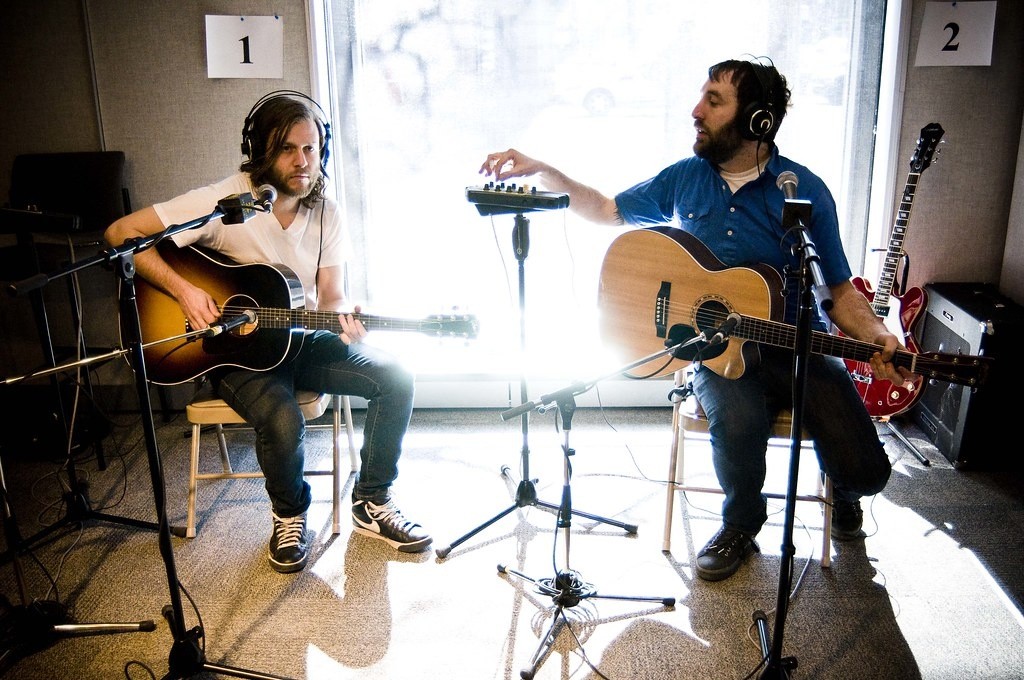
[437,207,835,680]
[0,190,296,680]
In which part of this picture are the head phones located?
[241,89,331,169]
[739,62,773,142]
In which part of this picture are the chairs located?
[662,367,833,569]
[186,389,356,538]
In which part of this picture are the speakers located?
[908,283,1024,470]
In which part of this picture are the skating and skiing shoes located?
[267,508,307,572]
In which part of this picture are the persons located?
[104,99,435,574]
[481,59,923,581]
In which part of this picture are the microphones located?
[205,310,257,338]
[258,184,278,213]
[711,312,742,346]
[775,171,813,230]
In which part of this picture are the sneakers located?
[351,493,433,553]
[817,469,863,540]
[695,525,760,581]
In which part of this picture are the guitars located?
[596,227,1000,392]
[832,121,946,423]
[116,244,480,387]
